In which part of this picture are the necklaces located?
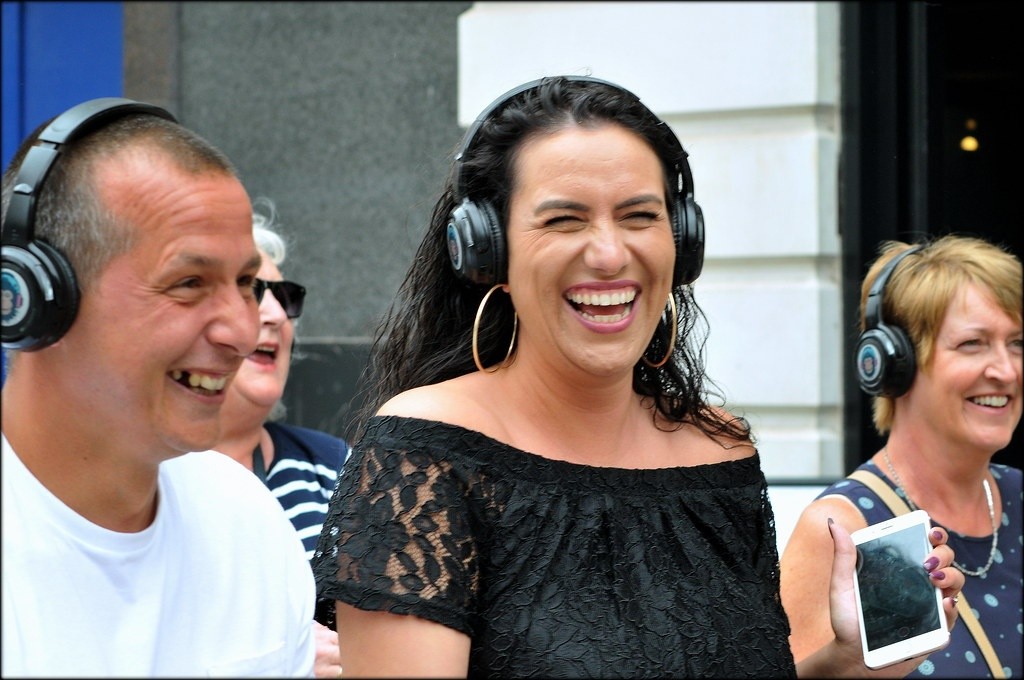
[883,445,998,576]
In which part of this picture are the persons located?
[205,197,354,677]
[0,111,316,678]
[778,232,1024,680]
[313,75,966,680]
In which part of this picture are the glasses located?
[252,278,306,318]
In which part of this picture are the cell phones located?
[851,509,950,671]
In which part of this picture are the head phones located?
[852,243,936,399]
[0,97,178,351]
[444,75,706,287]
